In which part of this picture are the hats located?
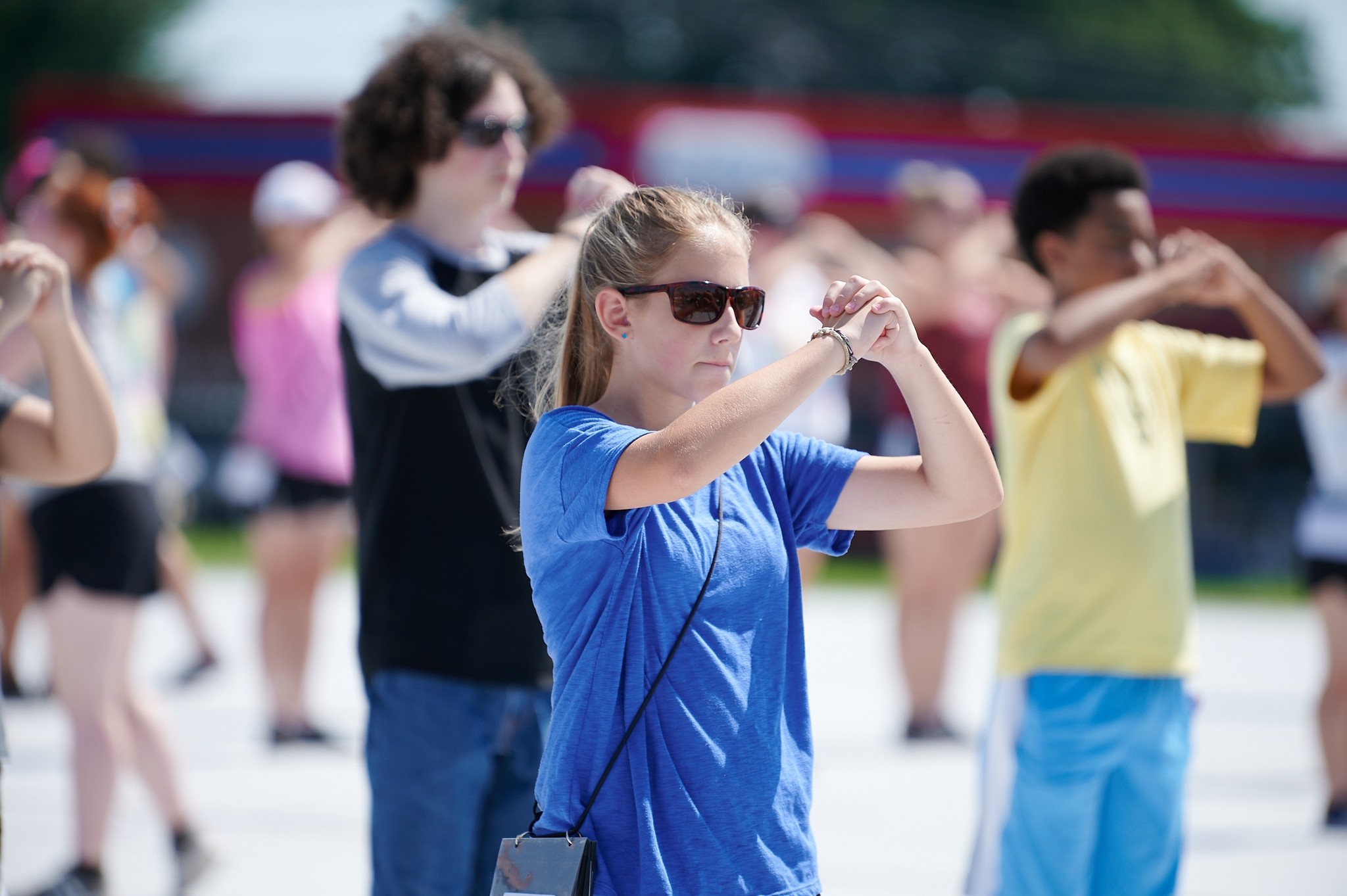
[251,162,339,229]
[1328,231,1347,276]
[19,137,55,183]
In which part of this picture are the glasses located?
[456,116,537,149]
[616,282,765,331]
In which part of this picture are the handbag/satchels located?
[489,828,596,896]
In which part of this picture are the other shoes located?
[907,719,958,739]
[172,841,214,896]
[45,686,52,696]
[29,871,104,896]
[1,681,22,698]
[177,657,217,684]
[269,727,324,745]
[1326,807,1347,826]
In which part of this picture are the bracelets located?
[810,325,855,378]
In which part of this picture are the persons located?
[0,19,1346,896]
